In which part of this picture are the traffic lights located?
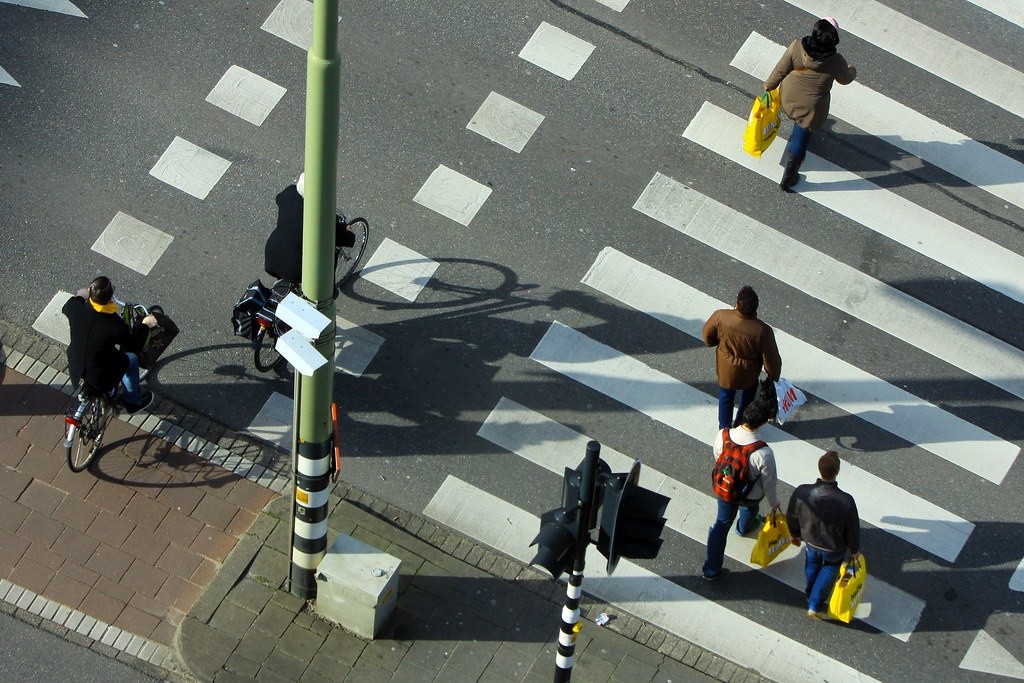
[596,458,671,577]
[527,506,581,584]
[561,456,613,531]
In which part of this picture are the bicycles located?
[61,290,165,473]
[242,210,371,373]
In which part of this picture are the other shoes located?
[703,569,728,581]
[126,390,154,414]
[808,609,822,620]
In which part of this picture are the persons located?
[61,276,158,416]
[702,399,781,581]
[265,173,356,299]
[702,285,782,430]
[764,17,857,189]
[786,451,860,622]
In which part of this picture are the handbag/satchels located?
[826,554,866,624]
[231,278,271,342]
[750,506,792,568]
[125,301,181,371]
[743,86,781,159]
[754,377,808,426]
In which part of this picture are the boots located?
[780,149,806,189]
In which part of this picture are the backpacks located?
[711,428,769,504]
